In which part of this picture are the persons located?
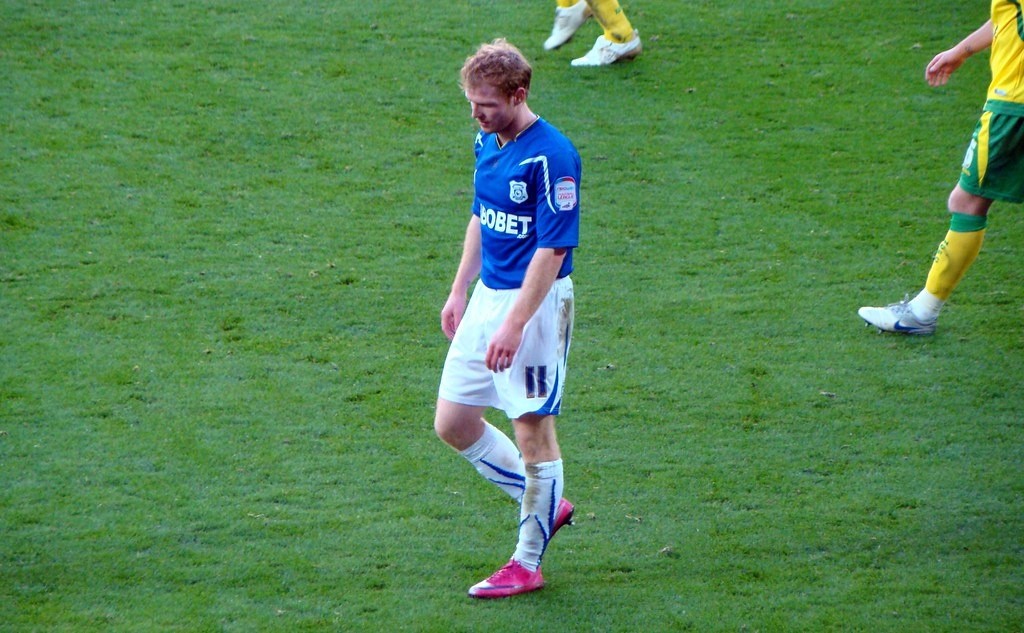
[434,37,582,599]
[858,0,1024,334]
[544,0,643,68]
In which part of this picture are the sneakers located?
[549,498,575,541]
[571,28,642,65]
[544,0,593,49]
[468,559,544,599]
[858,294,936,335]
